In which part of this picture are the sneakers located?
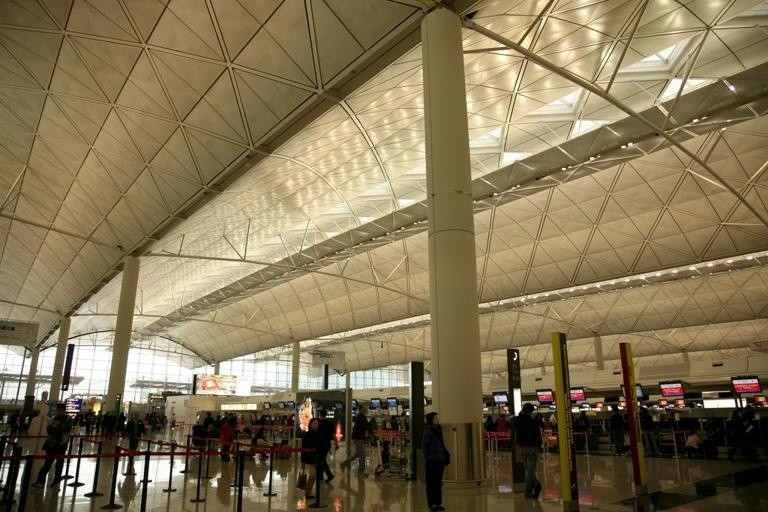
[526,483,541,499]
[306,495,316,499]
[32,479,60,488]
[320,474,334,483]
[428,505,445,512]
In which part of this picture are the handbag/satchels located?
[296,472,308,490]
[441,450,450,465]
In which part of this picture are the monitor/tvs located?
[424,397,428,406]
[277,402,285,409]
[570,387,587,402]
[536,389,554,403]
[287,401,295,409]
[334,401,344,411]
[352,399,358,410]
[370,397,382,409]
[492,390,508,405]
[731,375,763,394]
[387,397,398,409]
[620,383,644,399]
[658,380,686,398]
[264,402,271,409]
[312,400,320,408]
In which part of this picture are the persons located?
[317,408,339,483]
[483,404,768,462]
[421,411,452,511]
[391,415,399,444]
[511,496,544,512]
[300,417,323,500]
[544,458,716,500]
[115,461,143,510]
[368,415,378,448]
[31,492,63,511]
[16,408,29,436]
[400,412,410,445]
[339,403,373,477]
[29,401,72,491]
[187,454,291,508]
[312,478,410,511]
[327,419,336,463]
[71,407,294,460]
[510,402,542,501]
[7,407,20,435]
